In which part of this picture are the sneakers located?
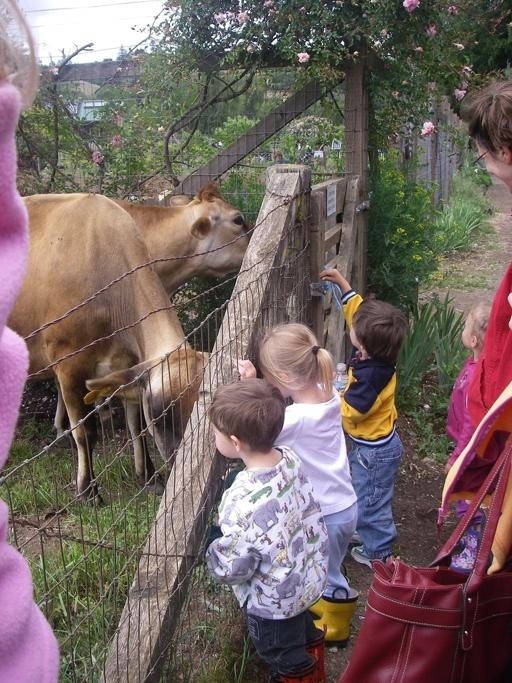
[349,528,391,571]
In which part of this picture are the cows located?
[7,191,211,507]
[53,184,255,450]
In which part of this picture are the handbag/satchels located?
[340,430,512,683]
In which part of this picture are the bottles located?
[335,361,349,389]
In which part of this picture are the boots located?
[277,628,328,682]
[313,594,359,646]
[307,593,324,617]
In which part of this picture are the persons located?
[237,320,360,649]
[444,297,496,573]
[317,266,409,573]
[203,376,330,679]
[454,77,512,574]
[0,0,62,680]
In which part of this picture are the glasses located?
[473,148,492,169]
